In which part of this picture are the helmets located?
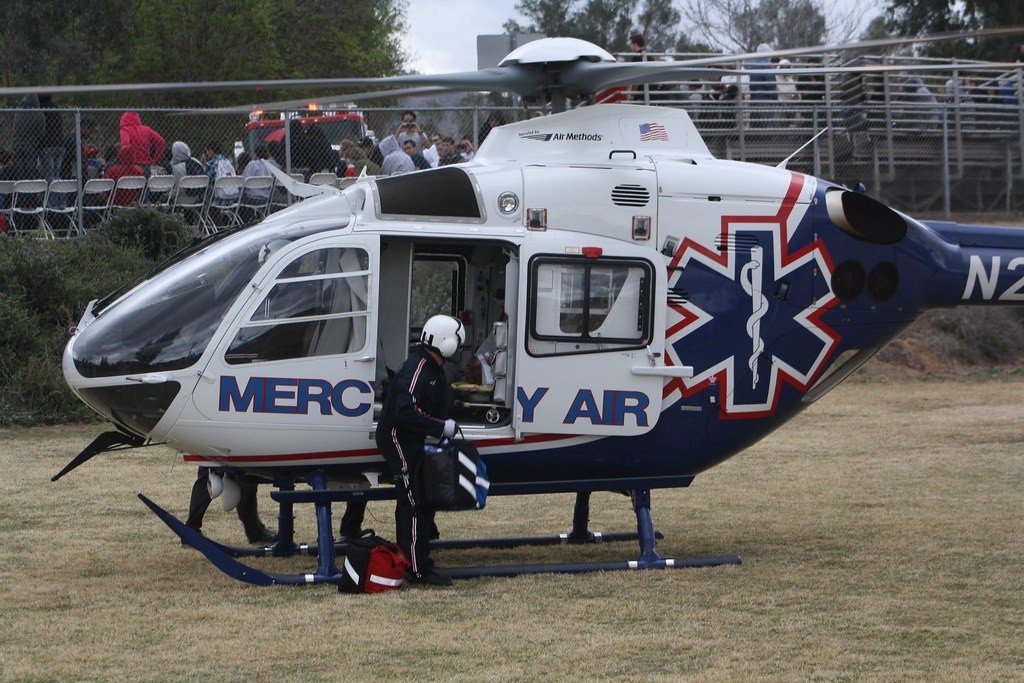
[421,315,466,358]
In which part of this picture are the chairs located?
[0,170,406,240]
[309,274,350,356]
[451,380,494,412]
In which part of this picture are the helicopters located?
[0,35,1024,585]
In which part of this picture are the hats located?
[757,42,773,54]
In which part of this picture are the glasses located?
[404,118,414,123]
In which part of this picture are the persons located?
[0,34,1024,223]
[375,315,466,585]
[184,464,279,544]
[340,500,438,539]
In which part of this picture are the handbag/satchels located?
[338,529,410,593]
[420,424,491,512]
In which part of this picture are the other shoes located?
[408,571,452,587]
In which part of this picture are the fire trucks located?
[244,102,380,177]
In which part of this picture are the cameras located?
[406,123,414,128]
[459,144,465,149]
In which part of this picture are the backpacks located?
[214,159,239,200]
[185,159,207,196]
